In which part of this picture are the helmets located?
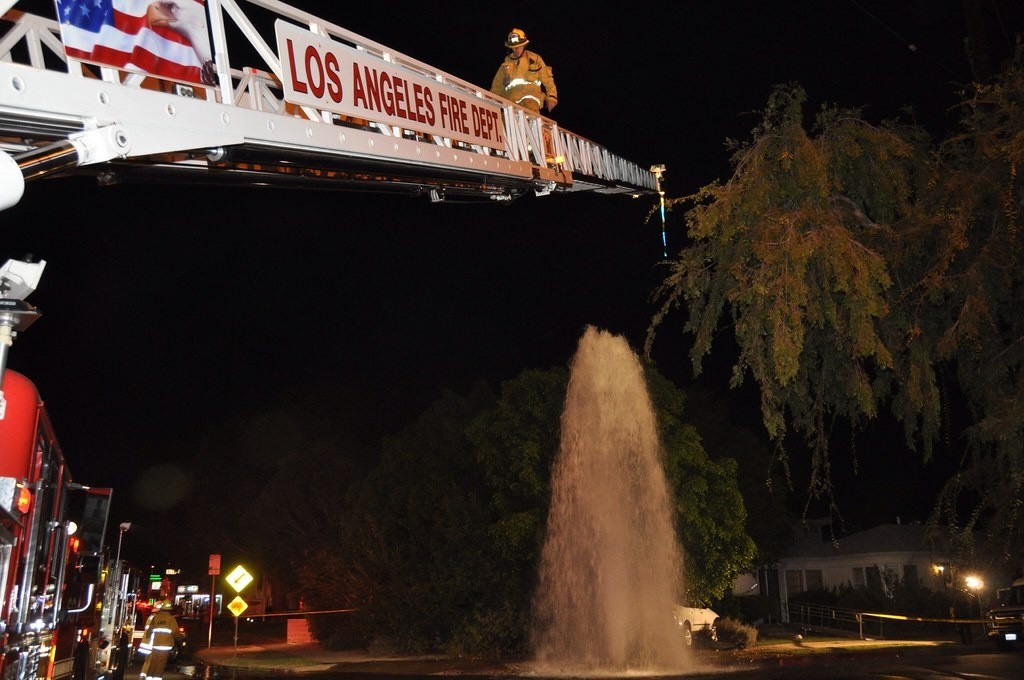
[151,601,163,613]
[161,600,174,610]
[504,28,529,47]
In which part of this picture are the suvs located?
[984,579,1024,652]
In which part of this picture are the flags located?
[52,0,215,90]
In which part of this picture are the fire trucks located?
[0,2,671,680]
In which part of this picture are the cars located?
[676,598,722,649]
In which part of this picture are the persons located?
[950,582,974,646]
[489,28,558,116]
[138,601,183,680]
[193,605,204,623]
